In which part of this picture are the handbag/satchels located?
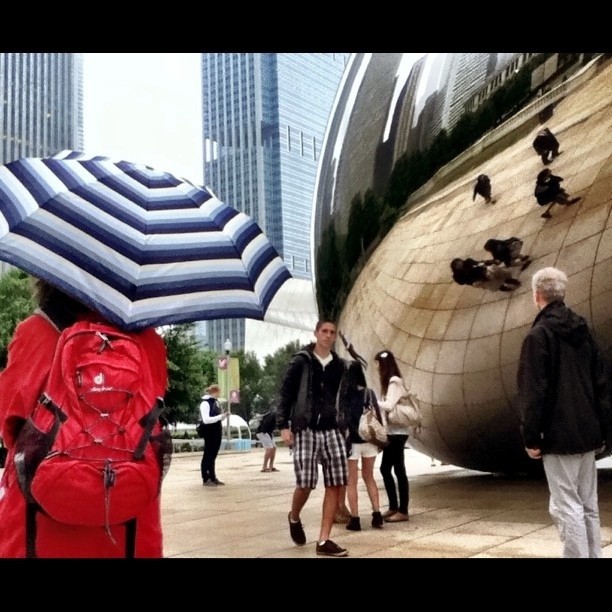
[387,392,423,429]
[358,388,390,450]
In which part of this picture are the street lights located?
[224,339,232,450]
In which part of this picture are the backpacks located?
[14,319,172,559]
[249,411,269,429]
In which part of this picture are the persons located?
[199,384,231,486]
[0,149,293,334]
[533,169,581,218]
[1,280,168,558]
[530,126,563,165]
[347,359,382,530]
[256,410,280,472]
[333,486,353,524]
[449,258,521,293]
[483,239,532,272]
[515,266,609,557]
[276,321,349,558]
[337,329,369,371]
[374,348,411,522]
[472,173,498,206]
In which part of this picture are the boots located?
[371,511,384,527]
[346,516,361,531]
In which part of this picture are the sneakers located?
[288,510,306,546]
[316,539,348,556]
[214,479,225,485]
[203,479,217,486]
